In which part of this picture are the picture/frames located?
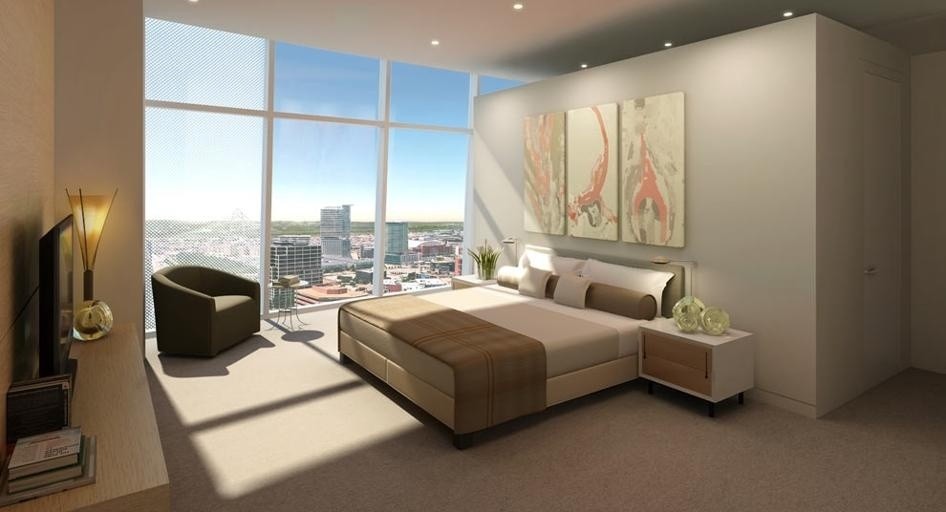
[622,92,685,249]
[523,111,565,237]
[566,103,618,242]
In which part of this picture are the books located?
[2,425,96,506]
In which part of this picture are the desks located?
[2,323,171,512]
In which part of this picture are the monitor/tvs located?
[37,215,78,394]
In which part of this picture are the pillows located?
[583,258,675,317]
[519,250,584,308]
[554,273,591,309]
[519,266,552,300]
[497,264,657,320]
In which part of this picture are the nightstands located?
[638,315,754,417]
[452,273,497,291]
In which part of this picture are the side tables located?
[268,279,309,331]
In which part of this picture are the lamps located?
[502,236,518,267]
[65,187,121,341]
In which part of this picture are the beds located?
[338,244,696,451]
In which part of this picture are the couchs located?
[151,263,261,359]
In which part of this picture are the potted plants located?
[465,238,504,280]
